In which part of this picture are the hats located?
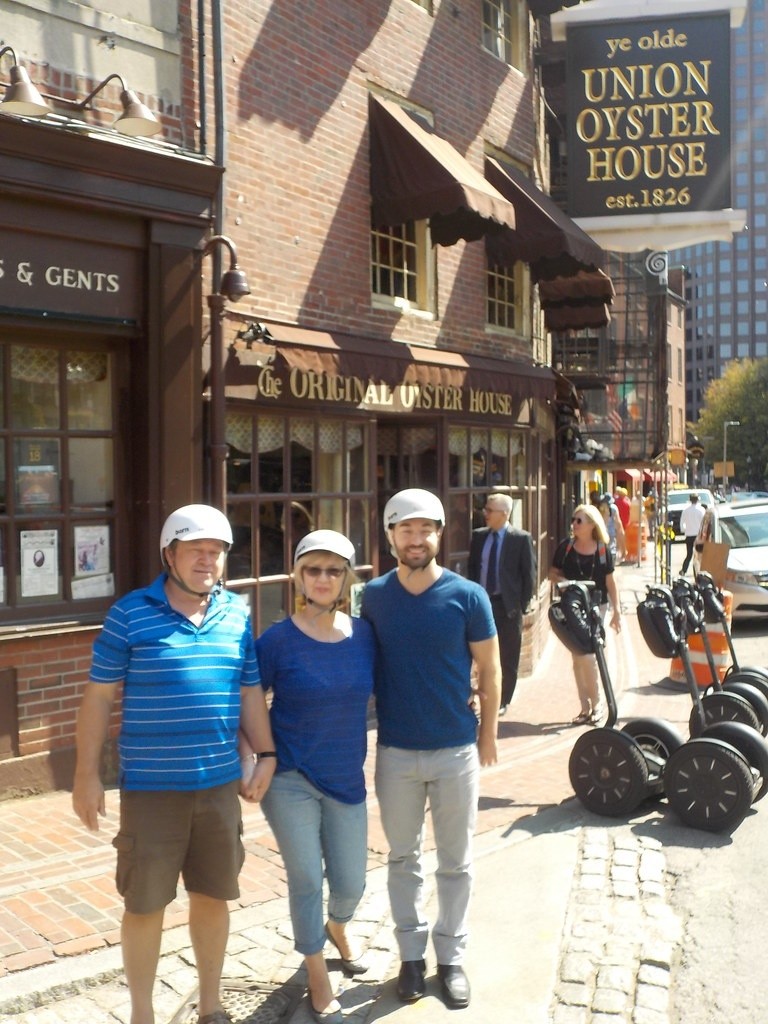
[690,494,699,500]
[617,487,627,496]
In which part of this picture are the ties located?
[486,531,499,593]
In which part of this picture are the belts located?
[489,594,503,599]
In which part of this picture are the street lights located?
[723,421,739,498]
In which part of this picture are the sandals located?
[588,709,604,724]
[571,714,592,724]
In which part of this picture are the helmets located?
[383,488,446,545]
[159,504,233,566]
[294,529,356,566]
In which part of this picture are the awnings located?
[367,90,615,331]
[618,468,680,484]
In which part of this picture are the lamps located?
[74,74,162,137]
[204,235,252,303]
[558,422,614,463]
[0,46,52,117]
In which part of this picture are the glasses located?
[302,566,346,578]
[570,517,588,524]
[481,508,505,513]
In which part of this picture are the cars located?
[693,499,768,620]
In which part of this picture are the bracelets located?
[239,753,254,762]
[256,751,278,758]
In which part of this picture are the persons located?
[361,489,502,1008]
[593,484,725,576]
[548,505,621,724]
[72,503,277,1024]
[468,493,539,716]
[278,503,316,572]
[239,529,481,1024]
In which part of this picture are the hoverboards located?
[636,582,768,833]
[670,579,768,740]
[548,580,686,818]
[692,570,768,698]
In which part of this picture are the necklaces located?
[573,545,595,581]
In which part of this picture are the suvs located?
[658,489,716,545]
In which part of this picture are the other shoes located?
[199,1012,233,1024]
[325,923,368,972]
[307,989,343,1024]
[499,704,505,715]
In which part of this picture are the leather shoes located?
[437,965,470,1007]
[397,959,427,1000]
